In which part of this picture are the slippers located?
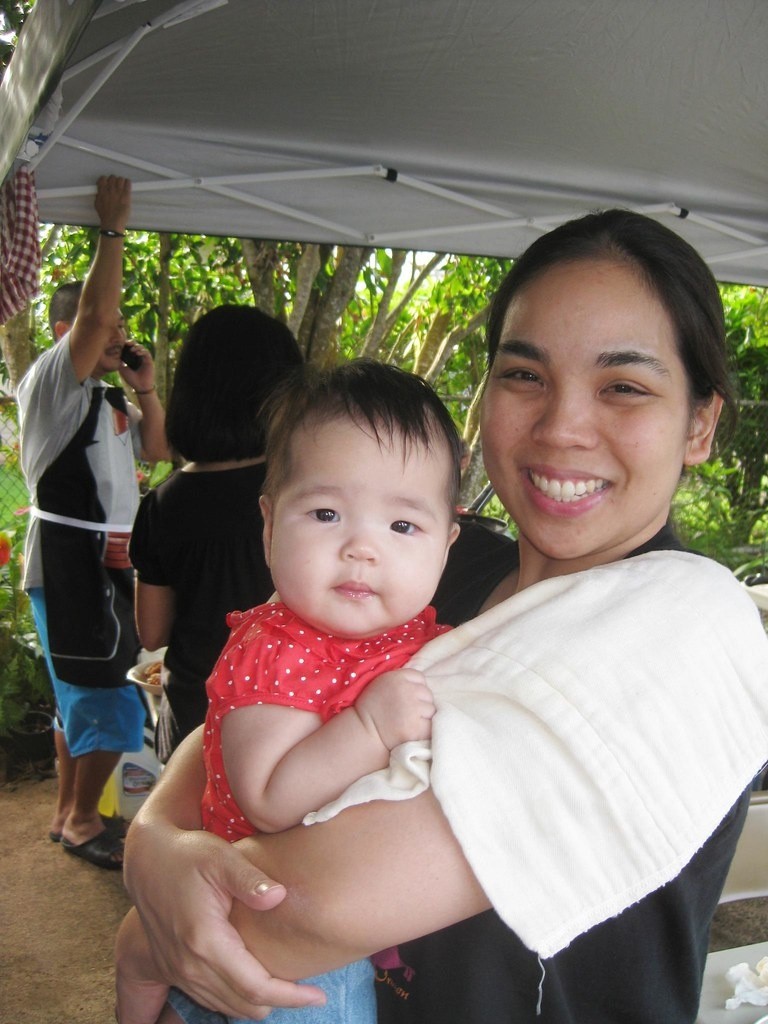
[50,815,128,842]
[61,832,125,866]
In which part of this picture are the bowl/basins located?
[126,660,164,696]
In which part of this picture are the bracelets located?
[100,230,126,238]
[135,386,155,394]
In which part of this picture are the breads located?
[145,661,162,687]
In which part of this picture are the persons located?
[13,175,169,870]
[129,302,305,769]
[114,208,768,1024]
[160,357,464,1024]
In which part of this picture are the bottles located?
[115,744,165,823]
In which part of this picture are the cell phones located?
[120,345,142,372]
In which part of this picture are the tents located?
[0,0,768,297]
